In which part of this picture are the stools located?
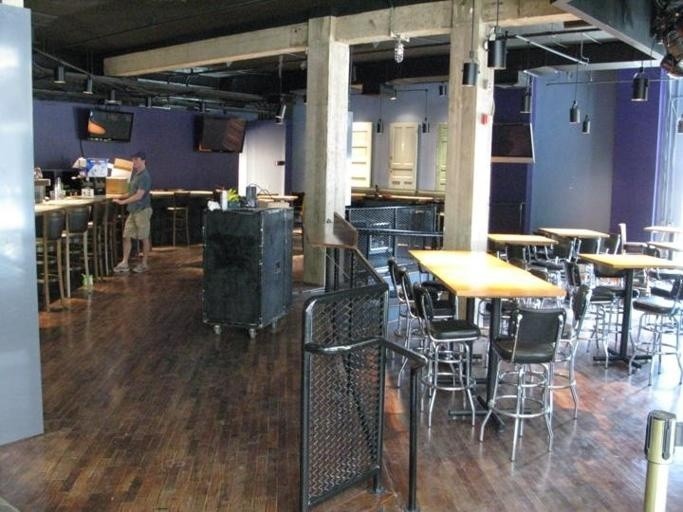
[386,260,593,461]
[489,222,683,386]
[37,192,304,311]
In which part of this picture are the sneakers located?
[131,263,151,273]
[113,263,130,273]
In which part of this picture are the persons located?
[111,151,153,273]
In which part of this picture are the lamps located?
[570,62,581,124]
[521,42,533,126]
[581,82,592,135]
[650,2,683,81]
[463,1,479,87]
[52,68,66,83]
[631,60,649,103]
[488,2,507,70]
[145,95,153,110]
[377,93,384,133]
[390,84,396,100]
[274,58,304,125]
[439,81,446,96]
[395,38,405,63]
[200,102,207,113]
[82,77,93,95]
[109,89,116,103]
[423,90,431,133]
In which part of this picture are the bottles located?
[213,189,227,212]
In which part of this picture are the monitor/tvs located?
[192,113,248,154]
[492,120,536,164]
[85,108,134,143]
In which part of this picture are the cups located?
[245,187,256,207]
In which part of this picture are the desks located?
[405,250,567,432]
